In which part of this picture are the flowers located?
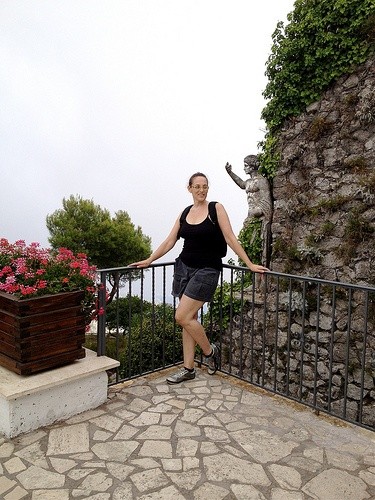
[0,237,109,330]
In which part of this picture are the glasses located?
[191,185,209,189]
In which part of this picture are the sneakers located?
[166,367,196,385]
[207,344,221,375]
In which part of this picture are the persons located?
[224,155,272,292]
[128,172,270,384]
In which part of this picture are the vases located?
[0,290,86,375]
